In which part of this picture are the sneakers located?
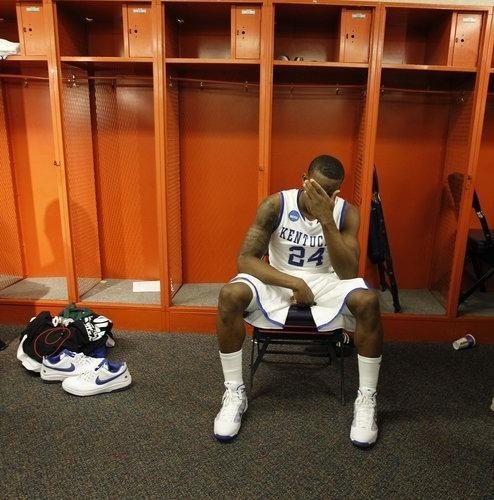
[40,349,107,384]
[214,382,248,441]
[62,358,133,397]
[350,389,378,448]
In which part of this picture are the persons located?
[213,152,383,451]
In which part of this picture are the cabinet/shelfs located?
[1,0,494,344]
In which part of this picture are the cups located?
[452,334,476,351]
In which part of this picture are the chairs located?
[246,307,354,405]
